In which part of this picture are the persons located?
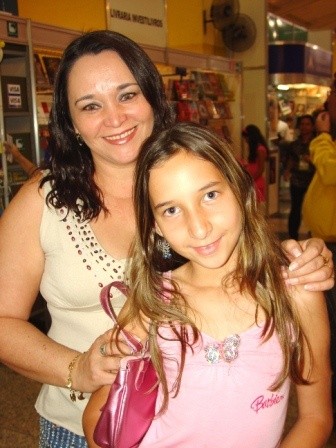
[3,140,38,179]
[0,29,335,448]
[236,81,336,251]
[81,122,333,448]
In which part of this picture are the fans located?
[203,0,239,35]
[221,14,256,57]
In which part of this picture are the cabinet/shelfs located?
[0,14,42,213]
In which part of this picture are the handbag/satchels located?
[88,281,159,448]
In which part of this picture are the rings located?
[100,344,109,358]
[320,254,328,266]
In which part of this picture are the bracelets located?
[67,353,85,402]
